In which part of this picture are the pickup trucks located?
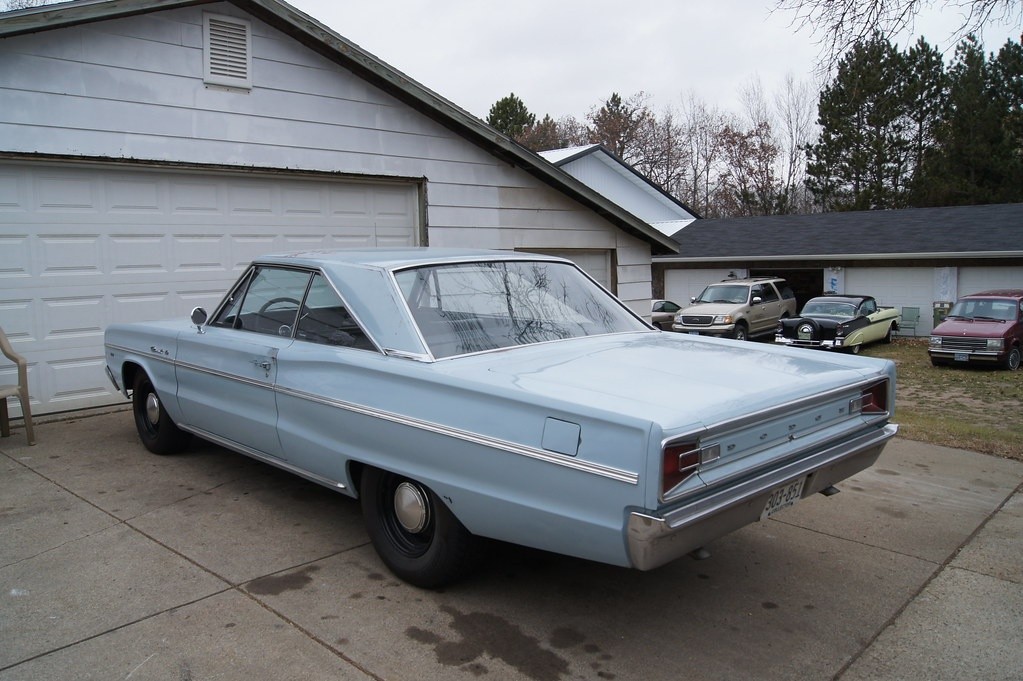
[774,293,903,355]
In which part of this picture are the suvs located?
[670,276,797,342]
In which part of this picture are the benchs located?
[303,308,482,340]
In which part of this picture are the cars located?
[927,286,1023,372]
[102,246,901,591]
[651,299,682,331]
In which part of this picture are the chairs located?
[973,304,989,315]
[0,324,37,446]
[894,307,920,339]
[1007,305,1015,318]
